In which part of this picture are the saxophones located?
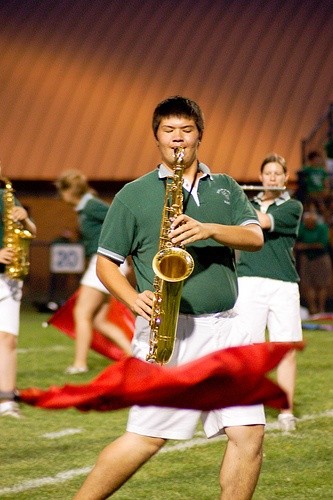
[0,177,32,280]
[146,146,194,367]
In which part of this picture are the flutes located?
[239,185,286,190]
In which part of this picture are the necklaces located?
[157,159,201,231]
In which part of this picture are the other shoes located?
[278,413,298,432]
[64,366,89,376]
[0,400,21,420]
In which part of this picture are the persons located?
[56,172,133,373]
[296,151,333,331]
[232,153,303,432]
[76,97,266,500]
[1,176,36,418]
